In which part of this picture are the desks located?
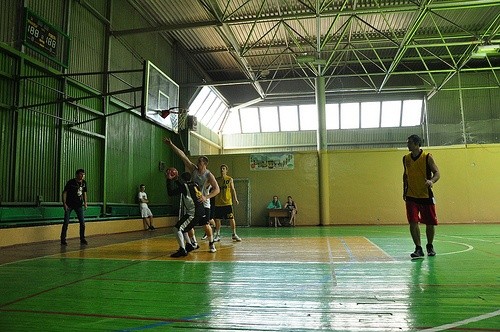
[268,208,289,227]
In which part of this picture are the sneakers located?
[214,235,220,242]
[411,247,424,257]
[148,227,155,231]
[426,245,436,256]
[61,240,67,246]
[209,242,216,252]
[191,242,200,249]
[81,239,88,244]
[184,243,194,253]
[232,234,241,241]
[201,233,208,240]
[171,247,188,257]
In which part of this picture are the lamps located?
[475,45,500,54]
[470,51,486,58]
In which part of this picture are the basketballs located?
[166,167,179,180]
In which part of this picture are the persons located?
[266,196,282,228]
[60,169,89,245]
[283,196,297,226]
[402,134,441,259]
[138,184,157,231]
[164,167,205,258]
[200,172,217,240]
[212,164,242,242]
[163,137,219,253]
